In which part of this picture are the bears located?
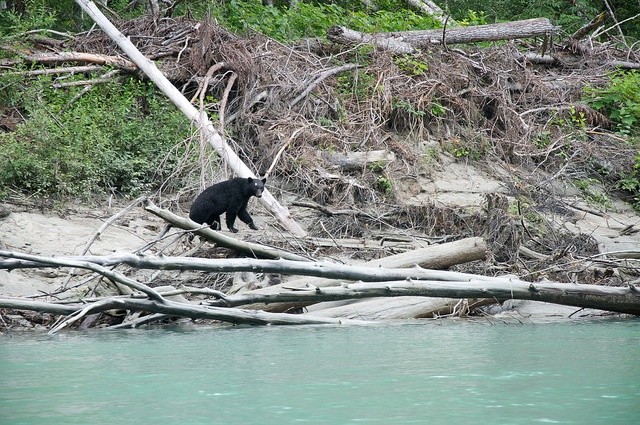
[190,178,267,242]
